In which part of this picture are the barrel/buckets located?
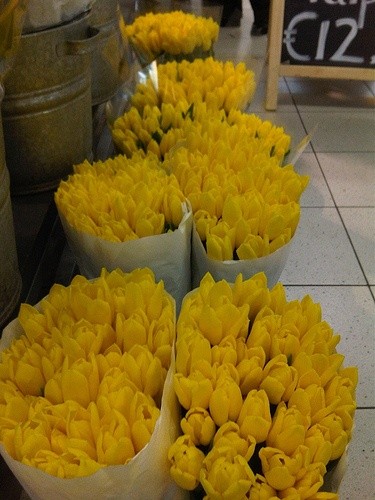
[1,0,116,325]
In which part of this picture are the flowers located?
[1,8,358,499]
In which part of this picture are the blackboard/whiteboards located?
[266,0,375,81]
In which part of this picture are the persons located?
[219,0,272,36]
